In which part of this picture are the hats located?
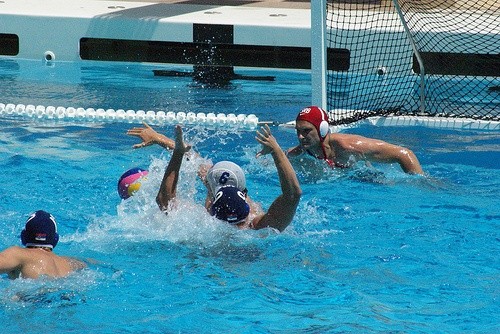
[207,161,246,197]
[296,106,327,140]
[21,210,58,251]
[210,185,249,224]
